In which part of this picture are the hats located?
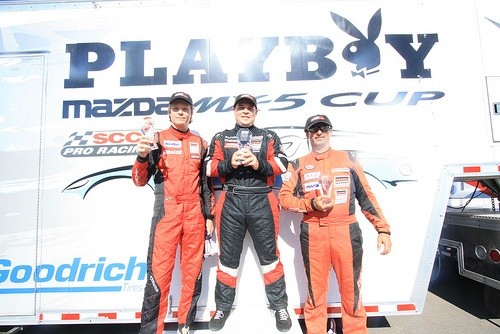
[304,114,333,131]
[233,93,257,110]
[169,92,193,106]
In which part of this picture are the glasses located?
[308,126,330,133]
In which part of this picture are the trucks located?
[0,0,500,334]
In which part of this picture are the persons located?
[278,115,391,334]
[132,92,215,334]
[202,94,292,332]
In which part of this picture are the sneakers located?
[177,325,190,334]
[208,309,231,330]
[276,309,292,332]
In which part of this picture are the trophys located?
[236,128,253,164]
[320,179,334,203]
[141,117,155,143]
